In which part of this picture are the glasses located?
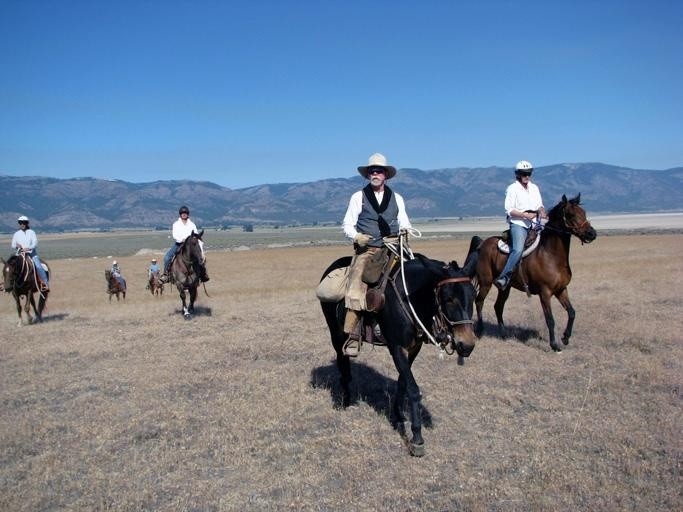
[519,172,531,177]
[367,166,384,175]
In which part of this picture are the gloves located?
[354,233,370,247]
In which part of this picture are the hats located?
[357,153,396,180]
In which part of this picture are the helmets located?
[179,206,189,214]
[18,215,28,221]
[516,160,533,171]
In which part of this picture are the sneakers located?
[346,339,358,354]
[496,276,506,287]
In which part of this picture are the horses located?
[319,251,479,457]
[146,268,164,296]
[464,191,597,352]
[105,268,126,303]
[169,229,206,321]
[0,255,51,327]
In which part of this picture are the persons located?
[494,161,547,290]
[145,259,164,290]
[107,261,127,293]
[340,152,412,358]
[158,206,209,284]
[3,216,49,293]
